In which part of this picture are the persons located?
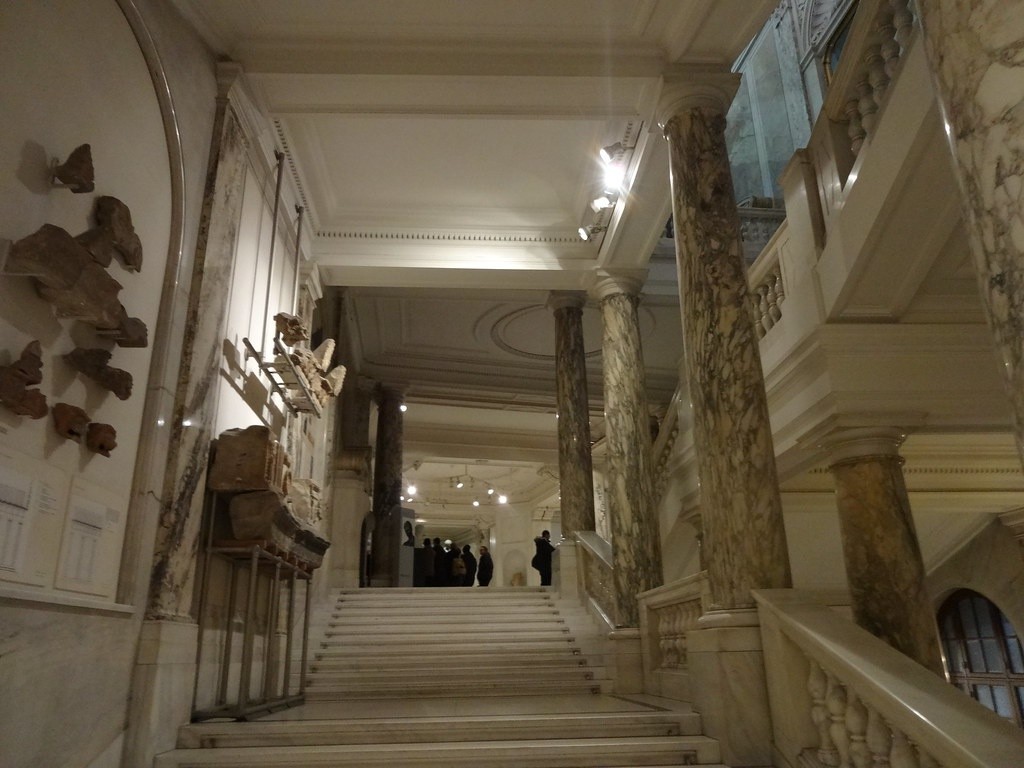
[423,537,493,587]
[534,530,556,585]
[403,520,415,546]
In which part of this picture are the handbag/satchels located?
[532,555,544,569]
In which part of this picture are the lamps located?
[449,477,454,487]
[487,495,493,503]
[472,496,480,506]
[425,497,431,506]
[599,141,634,163]
[589,197,617,213]
[456,476,464,488]
[486,483,495,495]
[604,187,615,195]
[578,223,607,241]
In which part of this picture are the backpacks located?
[451,556,467,577]
